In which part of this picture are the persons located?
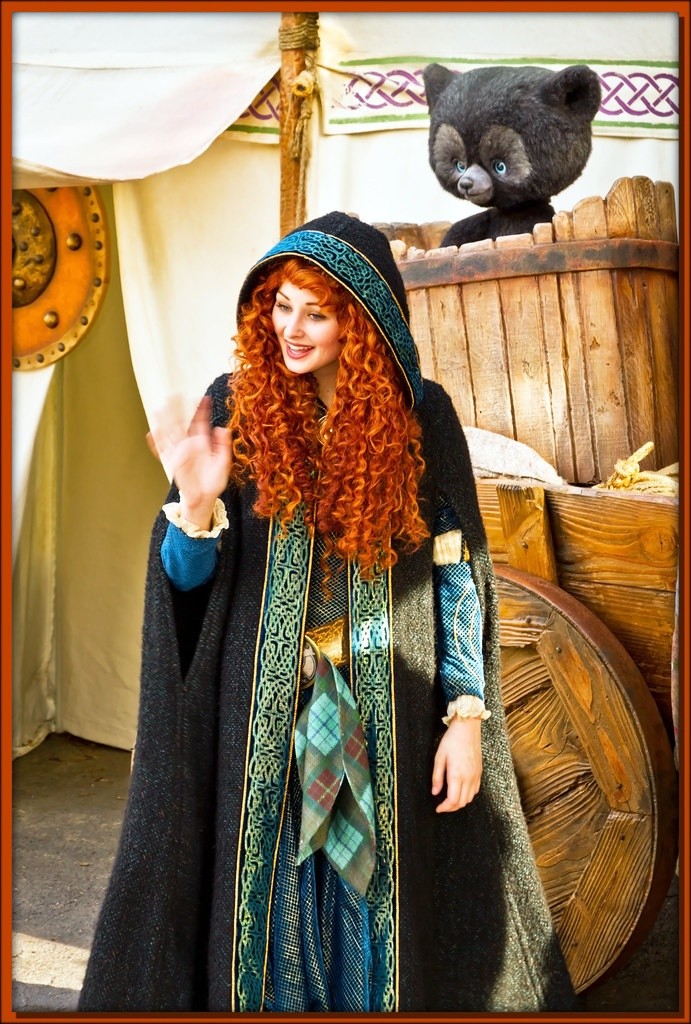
[75,211,575,1012]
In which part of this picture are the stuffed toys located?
[422,63,603,247]
